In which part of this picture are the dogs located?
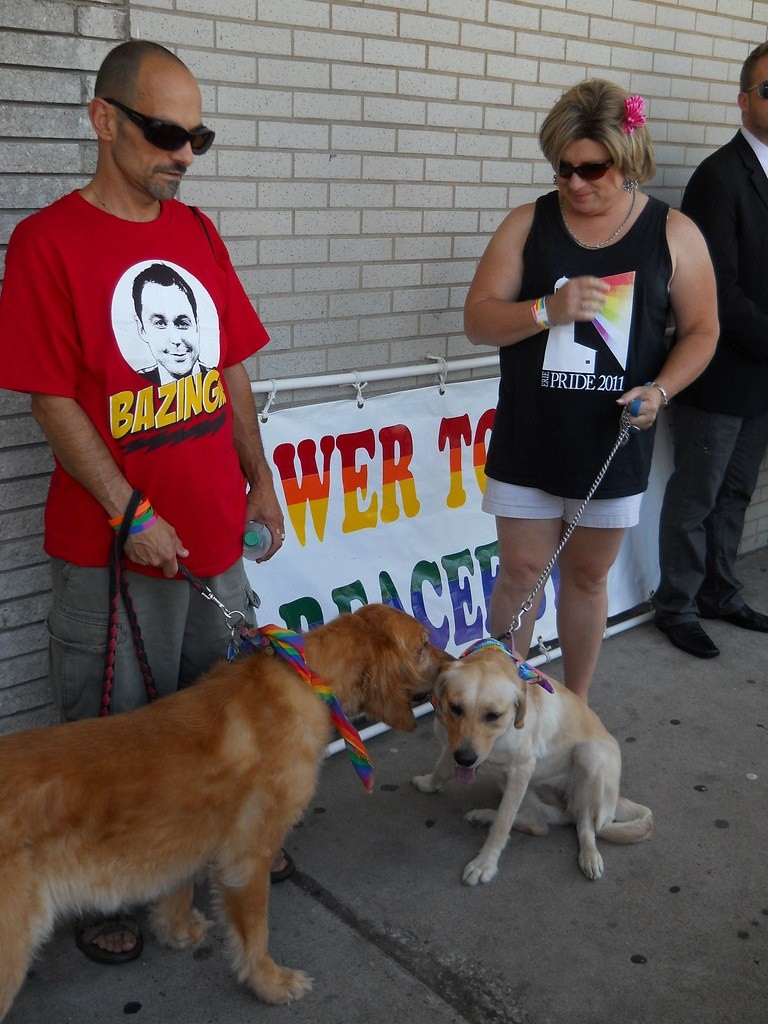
[413,645,656,888]
[0,602,462,1021]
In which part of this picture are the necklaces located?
[90,184,113,213]
[559,191,637,248]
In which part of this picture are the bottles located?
[241,519,273,561]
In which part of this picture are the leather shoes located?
[697,602,768,632]
[654,610,720,659]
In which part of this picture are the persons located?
[0,40,294,967]
[464,78,724,706]
[648,42,767,656]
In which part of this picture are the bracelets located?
[107,497,159,536]
[531,296,557,330]
[644,382,668,408]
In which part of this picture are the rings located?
[281,534,285,540]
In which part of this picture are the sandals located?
[269,846,296,884]
[73,908,144,965]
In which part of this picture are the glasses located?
[745,80,768,100]
[101,96,216,156]
[558,157,614,182]
